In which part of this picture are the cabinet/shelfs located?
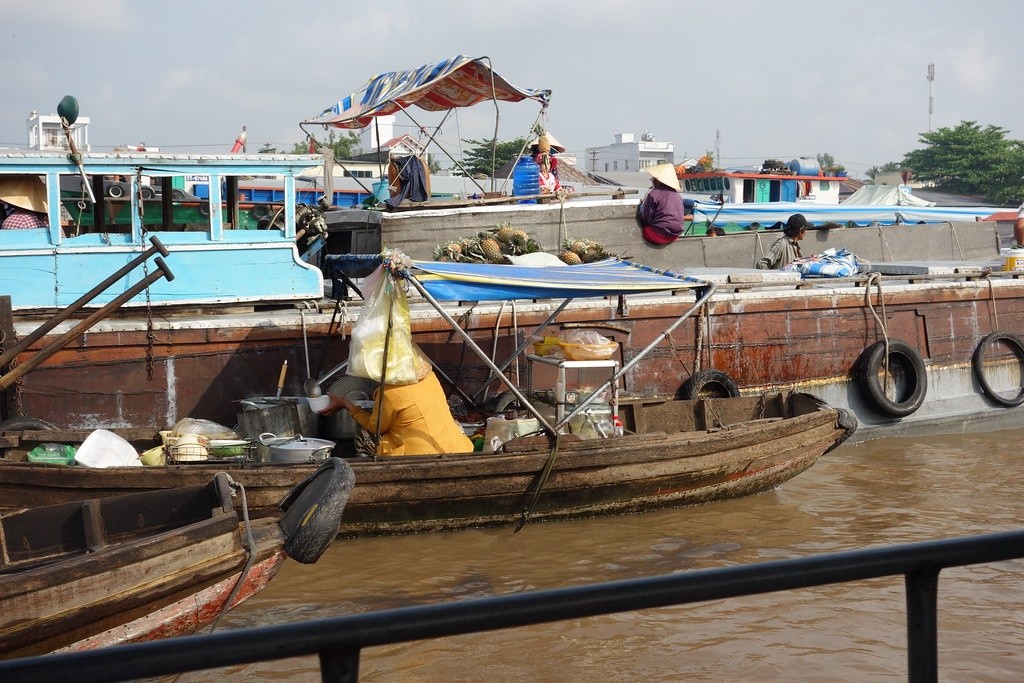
[526,352,621,439]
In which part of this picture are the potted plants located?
[824,164,848,177]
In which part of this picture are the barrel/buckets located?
[513,157,541,196]
[1001,248,1024,272]
[237,402,302,440]
[318,400,377,440]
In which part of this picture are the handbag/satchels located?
[346,267,433,385]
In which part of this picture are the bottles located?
[610,416,620,427]
[615,422,623,437]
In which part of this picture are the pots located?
[259,432,336,464]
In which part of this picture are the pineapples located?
[428,221,529,264]
[557,237,605,264]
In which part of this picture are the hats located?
[536,152,552,164]
[0,174,48,214]
[644,163,680,191]
[786,213,813,230]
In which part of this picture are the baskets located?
[159,430,251,468]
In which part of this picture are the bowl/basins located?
[138,446,165,465]
[173,433,250,461]
[306,395,331,414]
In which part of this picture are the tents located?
[840,185,936,207]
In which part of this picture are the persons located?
[706,227,725,237]
[638,163,685,245]
[318,371,474,457]
[756,214,814,272]
[528,129,565,193]
[0,174,67,237]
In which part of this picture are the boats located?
[0,455,357,662]
[1,385,860,539]
[0,143,1023,447]
